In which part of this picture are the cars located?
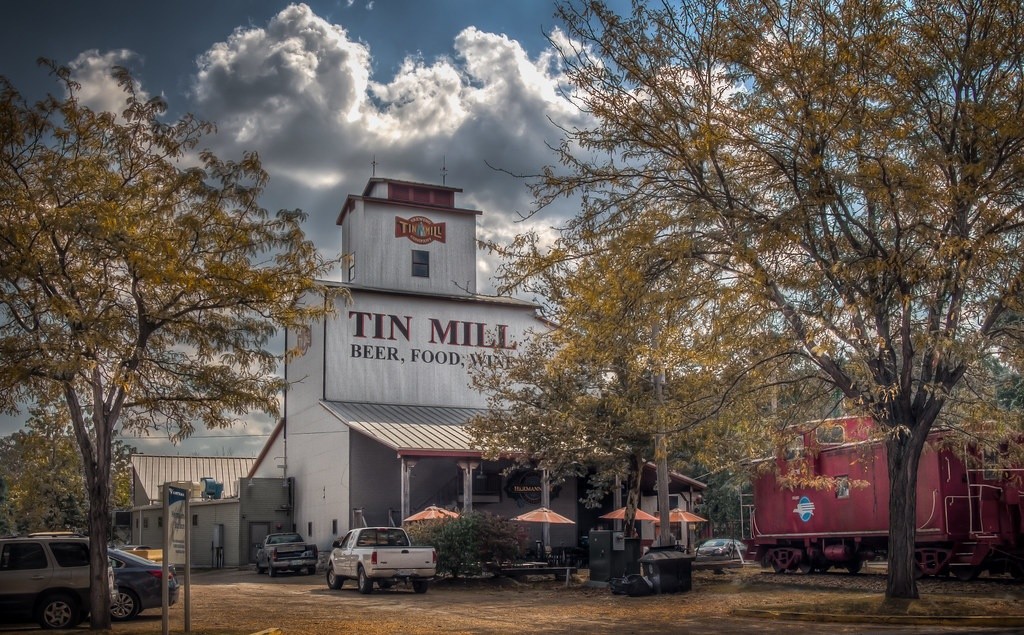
[107,545,179,622]
[696,538,746,559]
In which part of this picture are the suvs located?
[0,531,120,630]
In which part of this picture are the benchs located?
[501,567,577,587]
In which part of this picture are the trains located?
[746,415,1024,580]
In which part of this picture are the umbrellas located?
[403,503,710,554]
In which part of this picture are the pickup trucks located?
[255,532,319,576]
[324,526,437,595]
